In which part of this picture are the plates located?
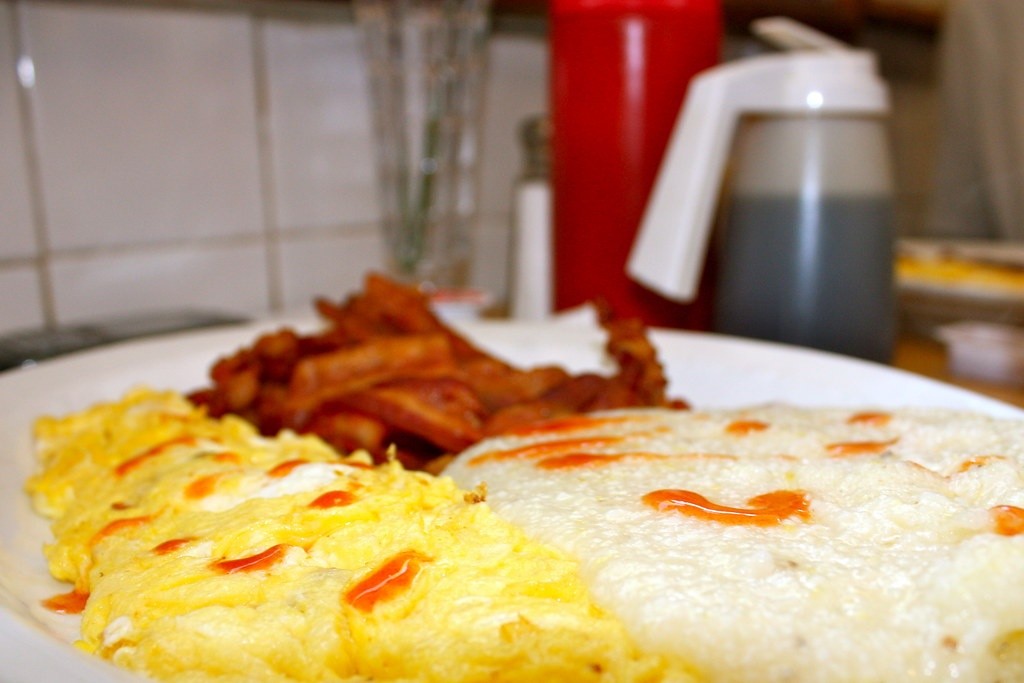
[1,315,1024,683]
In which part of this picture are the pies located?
[443,403,1023,683]
[29,387,716,683]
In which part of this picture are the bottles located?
[546,1,724,331]
[505,114,552,318]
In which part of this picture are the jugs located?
[626,14,899,365]
